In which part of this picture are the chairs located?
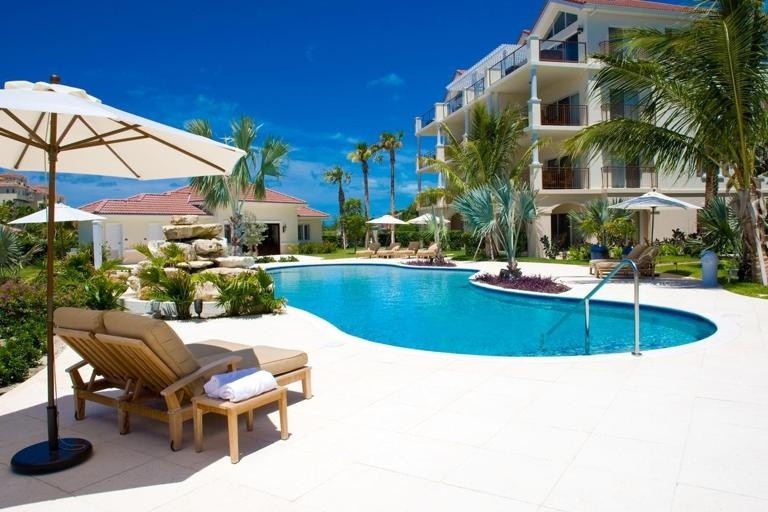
[355,241,438,259]
[543,169,572,189]
[588,243,658,281]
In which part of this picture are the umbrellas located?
[0,71,247,446]
[606,187,704,244]
[365,214,408,251]
[405,212,451,250]
[6,195,106,256]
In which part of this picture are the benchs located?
[53,305,312,452]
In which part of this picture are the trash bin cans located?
[701,251,719,288]
[591,247,608,259]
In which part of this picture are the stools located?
[190,385,290,462]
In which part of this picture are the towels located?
[201,366,279,403]
